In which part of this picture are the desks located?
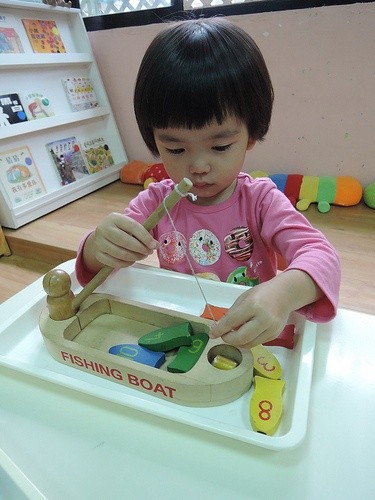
[0,256,375,500]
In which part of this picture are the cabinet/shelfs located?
[0,0,130,229]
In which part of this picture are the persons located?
[74,17,342,351]
